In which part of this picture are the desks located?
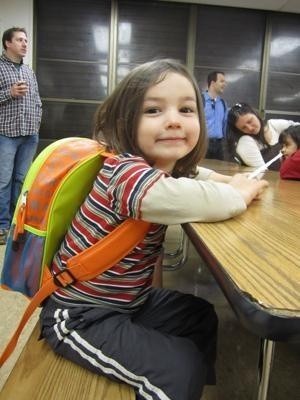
[161,173,300,400]
[163,158,273,260]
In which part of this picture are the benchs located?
[0,315,137,399]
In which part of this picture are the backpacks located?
[0,137,153,308]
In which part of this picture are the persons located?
[37,57,272,400]
[226,102,300,170]
[199,71,230,161]
[0,27,43,246]
[278,124,300,180]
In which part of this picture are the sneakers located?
[0,229,10,244]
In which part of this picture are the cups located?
[18,81,27,95]
